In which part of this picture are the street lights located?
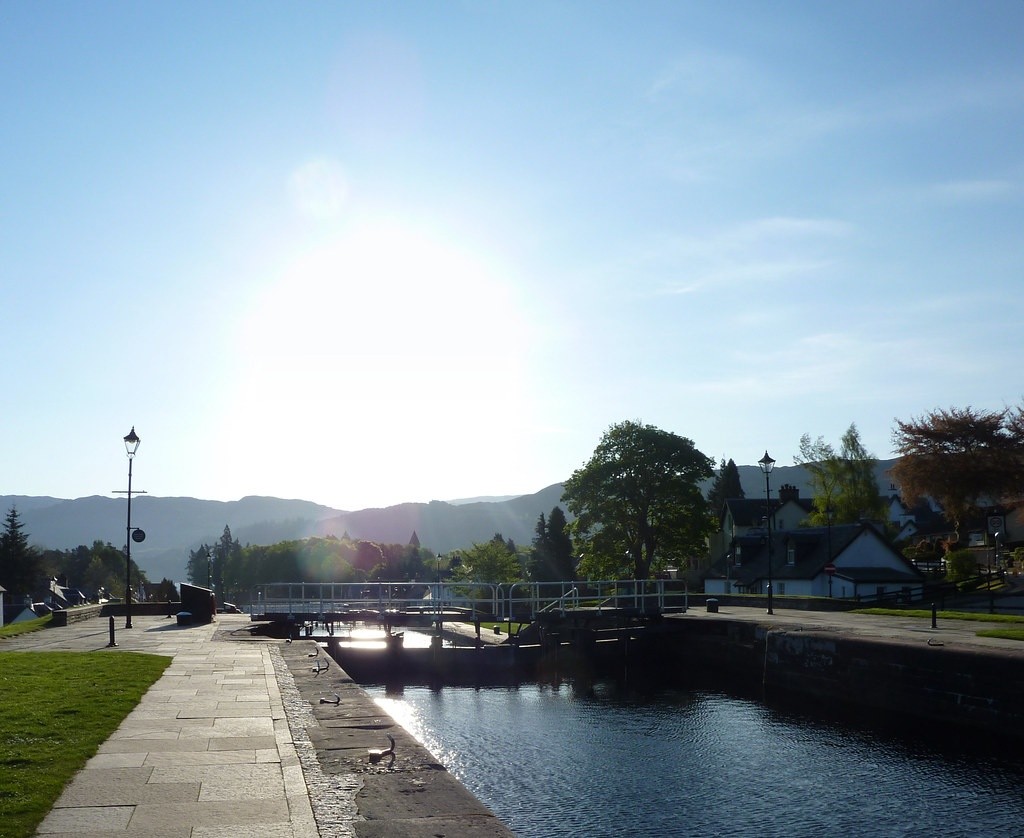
[757,450,777,616]
[436,552,443,614]
[206,552,211,588]
[123,426,142,628]
